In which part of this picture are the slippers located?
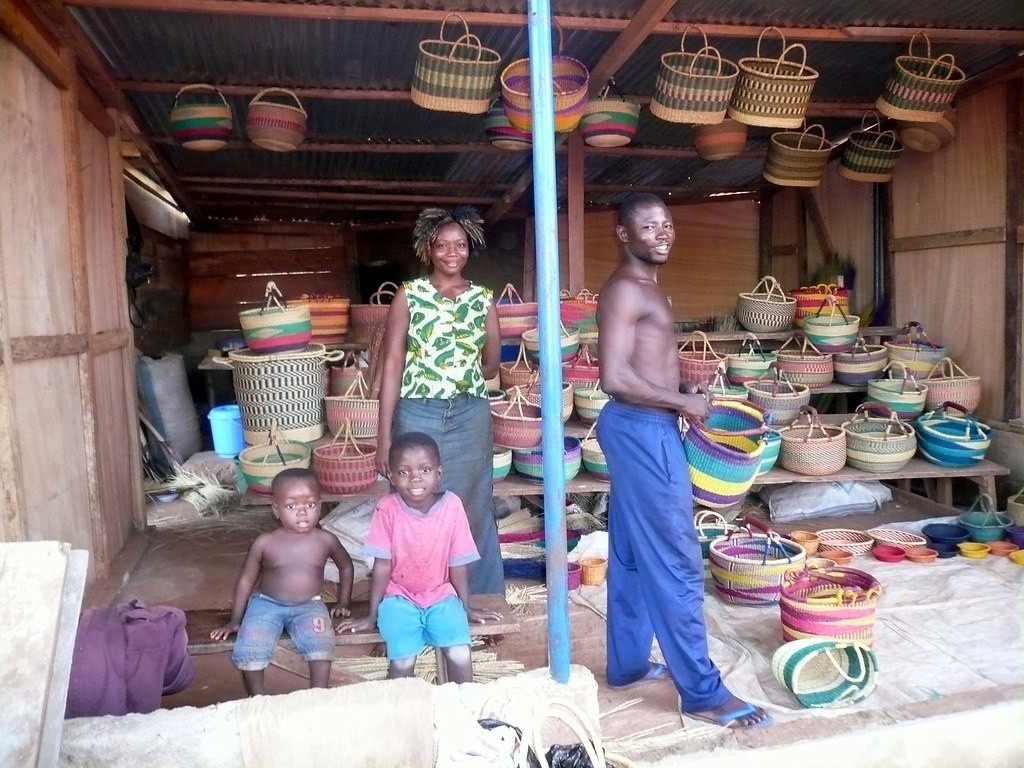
[606,660,673,691]
[682,688,773,730]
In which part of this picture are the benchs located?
[185,593,521,688]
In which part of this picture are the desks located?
[198,356,232,408]
[317,324,1014,519]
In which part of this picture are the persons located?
[210,468,355,698]
[368,207,506,657]
[596,194,774,728]
[334,433,504,684]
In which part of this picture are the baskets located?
[896,104,958,154]
[566,556,608,591]
[761,116,834,188]
[213,279,401,498]
[244,88,309,151]
[410,10,500,115]
[169,83,234,151]
[650,24,740,124]
[839,110,906,182]
[579,76,642,147]
[728,26,819,129]
[678,274,991,508]
[875,29,966,123]
[483,92,534,152]
[499,14,590,132]
[488,281,621,484]
[379,678,641,768]
[690,117,747,161]
[693,489,1024,710]
